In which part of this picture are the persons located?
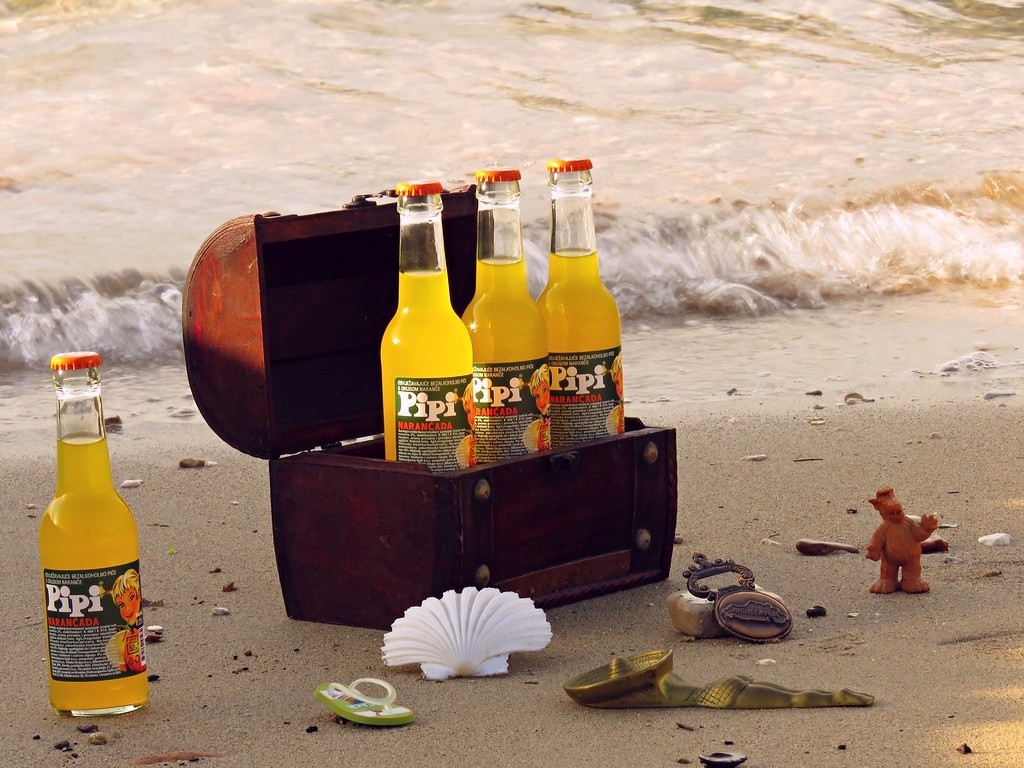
[564,649,874,709]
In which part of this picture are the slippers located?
[315,677,415,725]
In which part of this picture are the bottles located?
[378,158,622,469]
[40,351,148,717]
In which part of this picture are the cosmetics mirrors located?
[683,552,793,643]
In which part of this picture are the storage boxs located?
[183,184,679,630]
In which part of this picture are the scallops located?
[379,585,555,682]
